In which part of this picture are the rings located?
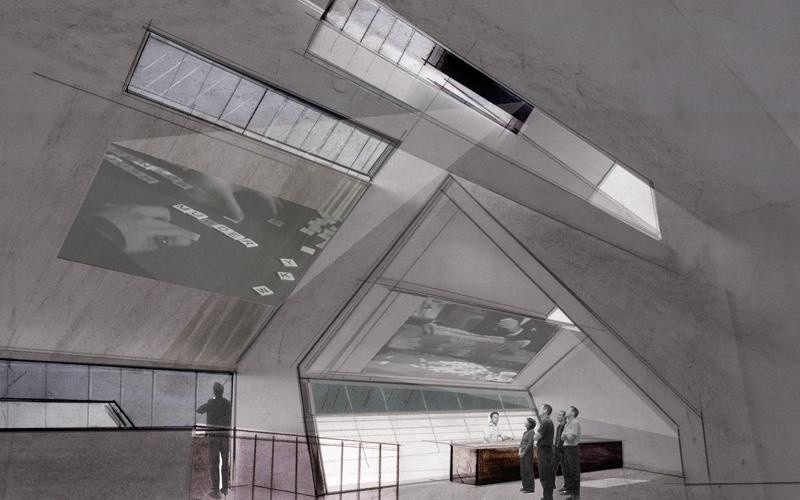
[154,235,169,248]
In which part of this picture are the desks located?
[417,432,625,487]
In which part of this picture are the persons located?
[58,167,285,259]
[561,405,581,500]
[533,403,554,500]
[196,381,232,499]
[482,411,513,443]
[469,312,556,352]
[552,410,567,491]
[518,418,537,493]
